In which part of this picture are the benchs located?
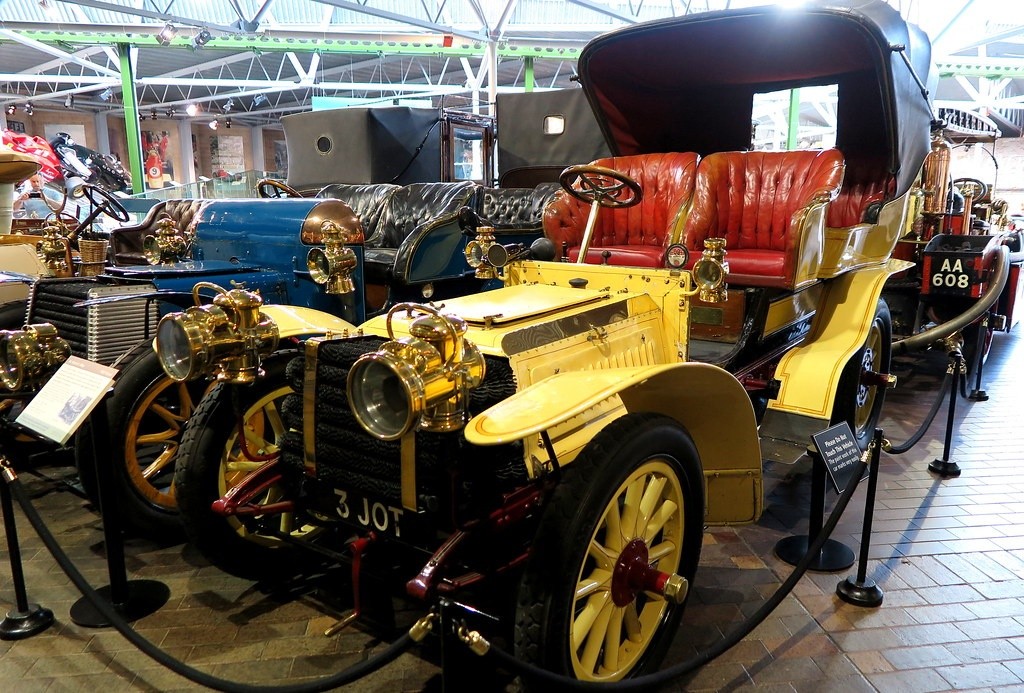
[481,182,562,234]
[314,180,484,284]
[823,151,897,228]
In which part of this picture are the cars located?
[0,1,1023,693]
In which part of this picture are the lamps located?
[208,118,218,131]
[149,107,158,119]
[222,97,235,110]
[191,25,210,50]
[98,88,113,101]
[157,20,177,47]
[64,93,75,108]
[226,117,231,128]
[166,105,176,120]
[5,105,17,115]
[22,101,34,116]
[252,94,267,106]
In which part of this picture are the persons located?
[462,144,474,177]
[13,172,45,210]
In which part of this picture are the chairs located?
[543,152,702,269]
[108,197,215,267]
[671,147,847,291]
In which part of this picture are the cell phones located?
[28,192,41,198]
